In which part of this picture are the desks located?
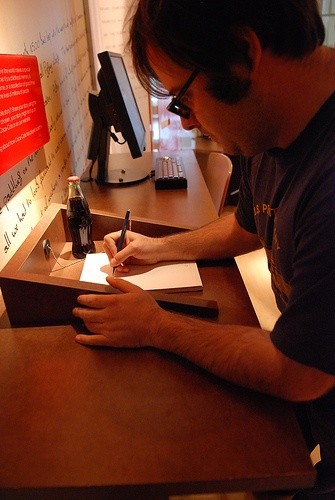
[0,150,317,500]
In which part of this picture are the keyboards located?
[154,156,187,189]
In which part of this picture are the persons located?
[73,0,335,499]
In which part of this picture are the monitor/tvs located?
[87,51,149,186]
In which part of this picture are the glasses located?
[166,70,199,120]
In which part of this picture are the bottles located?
[66,176,95,259]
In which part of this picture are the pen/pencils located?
[113,209,131,274]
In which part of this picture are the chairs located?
[205,152,233,215]
